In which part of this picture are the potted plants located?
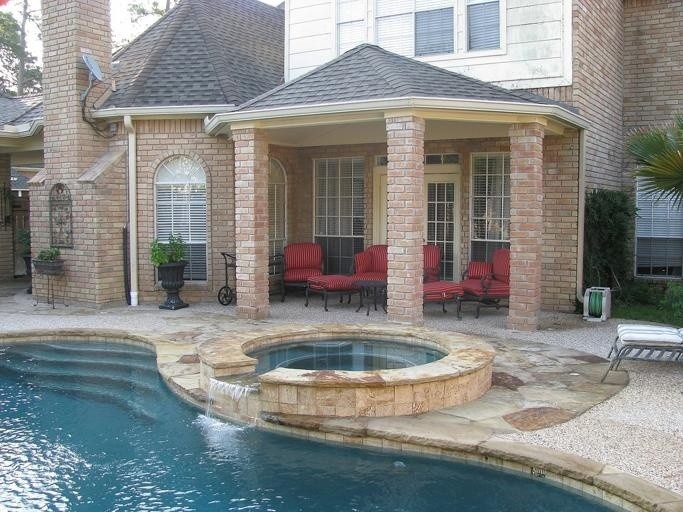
[32,248,65,276]
[15,228,31,295]
[148,232,190,311]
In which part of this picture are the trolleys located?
[217,252,283,306]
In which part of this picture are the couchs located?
[349,244,441,296]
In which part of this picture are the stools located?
[422,282,464,314]
[304,275,360,312]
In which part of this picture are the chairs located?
[277,242,324,302]
[601,324,682,381]
[459,248,509,318]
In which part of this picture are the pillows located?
[467,261,492,280]
